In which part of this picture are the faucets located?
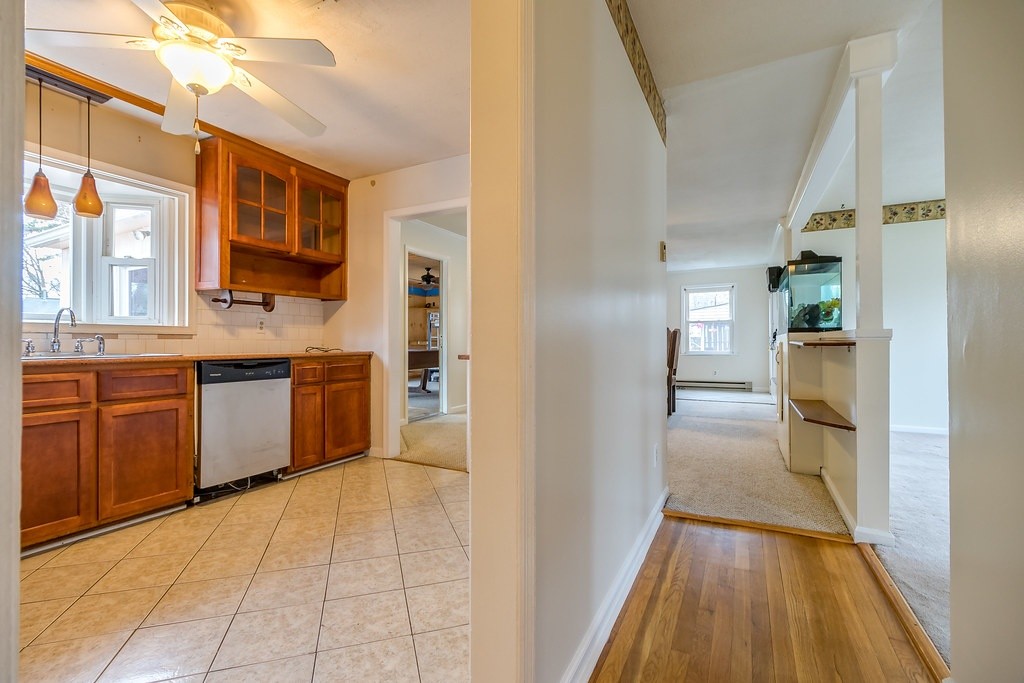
[50,307,76,352]
[95,335,105,356]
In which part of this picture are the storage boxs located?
[778,257,842,336]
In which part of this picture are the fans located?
[25,0,336,139]
[409,268,440,285]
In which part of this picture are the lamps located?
[72,95,104,219]
[155,41,235,155]
[421,284,434,291]
[24,79,57,220]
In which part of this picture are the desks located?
[408,349,439,394]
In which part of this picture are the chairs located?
[667,327,681,416]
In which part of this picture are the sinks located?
[21,350,182,359]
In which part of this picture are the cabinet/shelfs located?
[323,358,371,464]
[93,367,194,528]
[287,357,322,473]
[194,135,350,302]
[20,371,94,548]
[776,331,821,475]
[789,340,856,431]
